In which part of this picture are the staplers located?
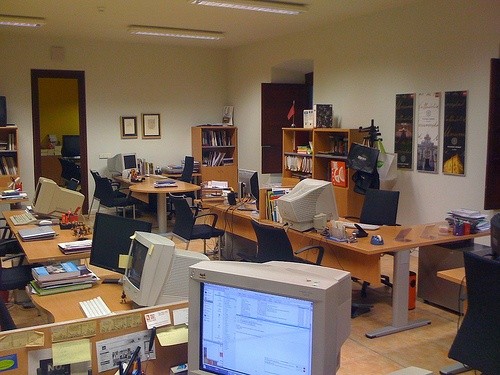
[353,223,368,237]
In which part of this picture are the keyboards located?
[10,213,36,225]
[79,296,111,318]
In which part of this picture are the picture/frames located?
[141,112,161,138]
[120,115,137,138]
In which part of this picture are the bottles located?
[453,220,476,236]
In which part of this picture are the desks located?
[0,174,491,375]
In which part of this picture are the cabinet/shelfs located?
[0,127,19,194]
[282,127,370,223]
[192,126,238,198]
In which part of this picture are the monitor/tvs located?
[237,168,259,203]
[277,178,339,232]
[89,212,153,273]
[107,153,138,174]
[122,231,210,307]
[188,259,352,375]
[32,177,85,218]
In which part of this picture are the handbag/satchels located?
[346,138,380,173]
[351,170,379,190]
[373,140,397,180]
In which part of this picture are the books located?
[285,141,313,175]
[30,261,101,296]
[0,133,17,176]
[265,188,293,223]
[137,159,156,177]
[201,130,235,166]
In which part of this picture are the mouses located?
[39,219,53,225]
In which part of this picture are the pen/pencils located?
[119,346,141,375]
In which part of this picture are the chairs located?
[448,249,500,375]
[179,156,194,183]
[98,175,137,220]
[249,216,324,266]
[87,169,121,220]
[57,158,81,187]
[359,189,401,226]
[0,254,45,309]
[0,218,21,257]
[169,192,225,261]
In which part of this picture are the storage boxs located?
[62,212,79,223]
[330,160,348,188]
